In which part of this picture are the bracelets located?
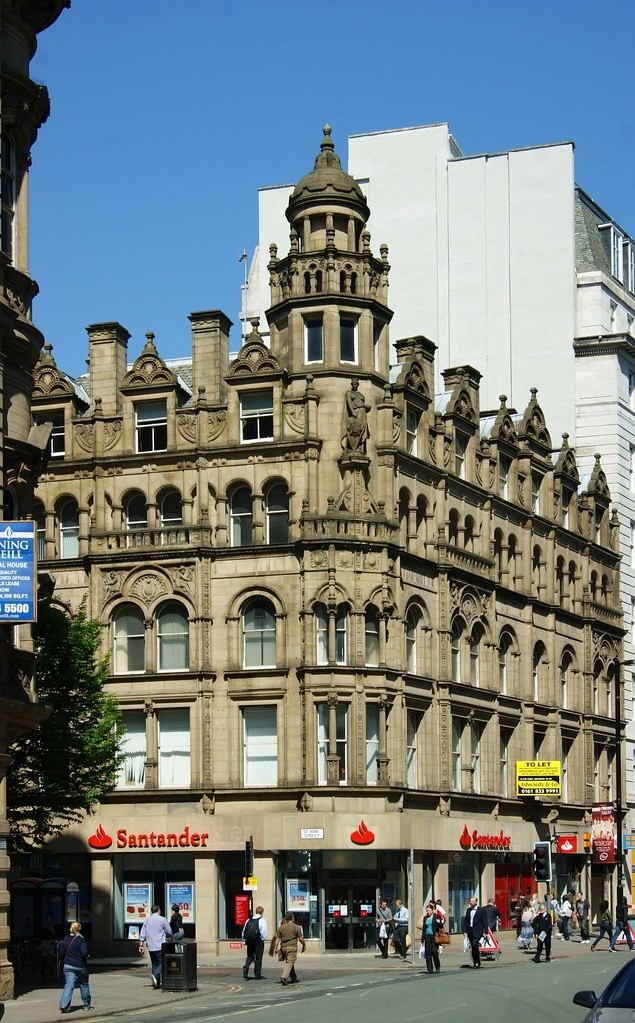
[139,945,143,947]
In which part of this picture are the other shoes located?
[243,968,248,979]
[545,958,550,963]
[631,947,635,951]
[609,947,617,952]
[60,1005,72,1013]
[291,979,300,983]
[280,978,289,986]
[532,958,540,963]
[399,956,408,962]
[255,976,264,979]
[83,1006,94,1011]
[591,945,595,952]
[436,967,440,974]
[426,970,433,974]
[380,953,388,960]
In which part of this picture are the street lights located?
[613,657,635,915]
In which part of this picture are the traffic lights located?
[533,842,553,883]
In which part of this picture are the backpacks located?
[243,917,262,942]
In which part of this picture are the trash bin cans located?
[161,940,197,992]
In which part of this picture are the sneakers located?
[580,939,591,944]
[150,974,162,989]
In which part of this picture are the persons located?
[516,892,561,962]
[103,571,120,600]
[421,899,447,974]
[594,634,599,651]
[557,891,590,944]
[60,922,95,1011]
[376,900,409,959]
[590,896,635,953]
[482,594,489,614]
[464,897,501,972]
[345,380,367,418]
[615,640,620,658]
[451,583,459,612]
[177,566,193,593]
[242,906,268,979]
[138,904,184,989]
[273,912,307,985]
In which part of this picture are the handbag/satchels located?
[277,949,286,961]
[405,934,413,948]
[387,924,394,933]
[379,922,387,938]
[418,941,426,960]
[433,926,450,945]
[462,934,472,953]
